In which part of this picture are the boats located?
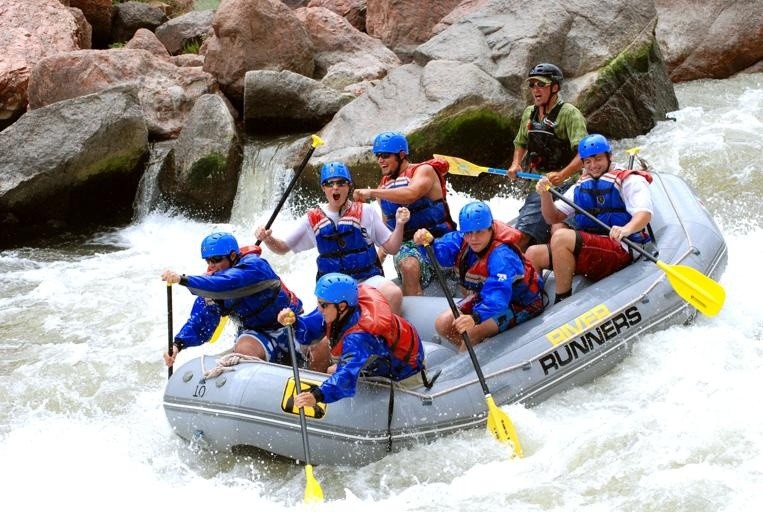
[163,171,727,468]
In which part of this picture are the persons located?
[414,199,545,354]
[256,162,411,315]
[276,272,427,409]
[508,62,588,251]
[163,231,304,368]
[353,131,456,296]
[523,133,654,304]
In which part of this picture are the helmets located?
[200,232,240,259]
[373,130,409,154]
[459,201,493,233]
[314,272,358,307]
[578,134,612,160]
[526,63,565,83]
[320,161,353,182]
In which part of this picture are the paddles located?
[544,185,727,317]
[418,233,525,459]
[284,312,325,504]
[208,134,325,345]
[431,153,549,180]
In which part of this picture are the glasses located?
[204,257,228,263]
[316,301,334,308]
[528,81,551,87]
[321,181,349,188]
[376,153,394,158]
